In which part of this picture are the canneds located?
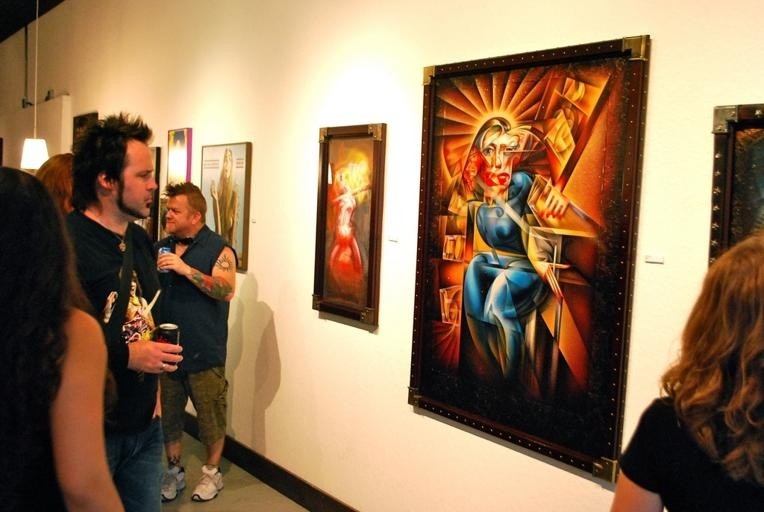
[158,323,180,366]
[156,247,171,274]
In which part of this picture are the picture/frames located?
[311,122,387,327]
[405,29,650,485]
[201,141,251,271]
[708,101,764,272]
[166,127,192,186]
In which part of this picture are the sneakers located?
[160,464,186,502]
[190,463,225,502]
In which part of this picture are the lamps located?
[20,0,52,172]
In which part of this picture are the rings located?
[161,364,165,369]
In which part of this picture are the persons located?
[34,153,74,220]
[1,165,125,512]
[63,112,183,511]
[153,182,238,502]
[612,229,764,512]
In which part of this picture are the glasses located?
[169,237,194,246]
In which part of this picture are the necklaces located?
[173,236,194,246]
[91,209,129,252]
